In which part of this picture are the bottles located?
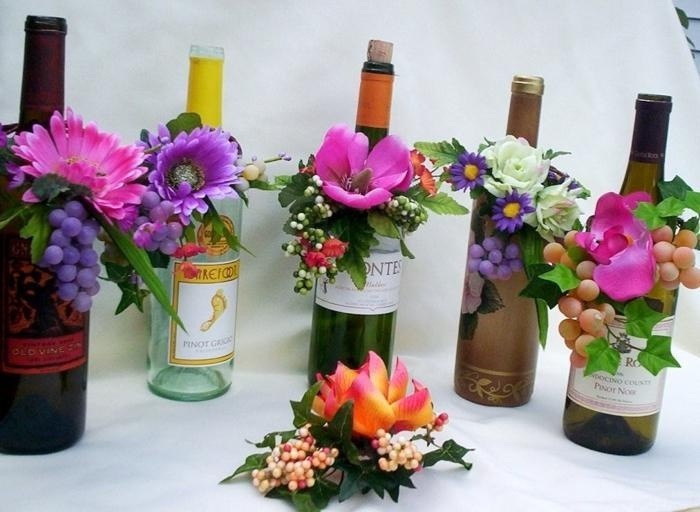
[305,40,405,396]
[452,73,547,408]
[1,14,91,457]
[143,43,245,405]
[560,93,691,458]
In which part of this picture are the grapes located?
[541,230,615,367]
[38,199,101,315]
[232,160,269,191]
[650,225,699,290]
[133,189,184,256]
[467,235,521,282]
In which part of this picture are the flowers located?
[119,120,246,286]
[220,350,476,512]
[0,107,151,316]
[448,133,590,278]
[276,125,468,298]
[543,176,700,376]
